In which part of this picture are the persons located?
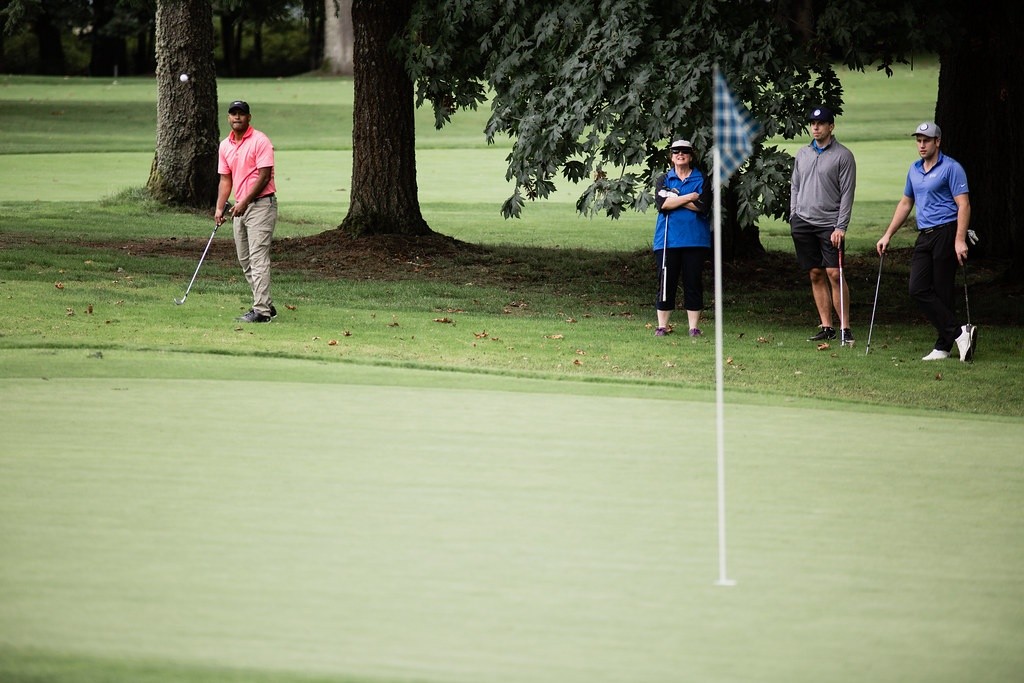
[214,101,278,323]
[652,134,711,337]
[876,122,978,361]
[789,108,857,342]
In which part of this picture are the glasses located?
[672,148,691,153]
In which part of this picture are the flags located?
[714,69,760,188]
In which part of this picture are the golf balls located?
[180,74,188,82]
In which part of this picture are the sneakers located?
[235,311,271,324]
[244,306,277,318]
[955,324,978,361]
[811,327,837,341]
[840,328,856,342]
[922,349,950,360]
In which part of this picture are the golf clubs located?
[858,254,884,357]
[661,188,680,302]
[962,260,974,364]
[839,244,847,347]
[173,202,229,305]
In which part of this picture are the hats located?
[228,101,249,113]
[911,122,941,139]
[669,140,693,148]
[810,107,834,123]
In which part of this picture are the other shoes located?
[689,328,700,337]
[654,327,666,335]
